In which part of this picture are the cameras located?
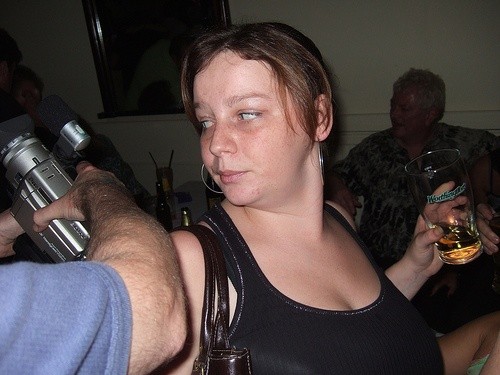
[0,120,92,263]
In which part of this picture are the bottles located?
[206,172,225,209]
[181,207,191,227]
[155,182,173,232]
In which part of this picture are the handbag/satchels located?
[168,225,252,375]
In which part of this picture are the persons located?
[323,68,500,375]
[0,27,188,375]
[145,21,468,375]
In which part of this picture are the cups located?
[156,167,173,200]
[405,149,483,265]
